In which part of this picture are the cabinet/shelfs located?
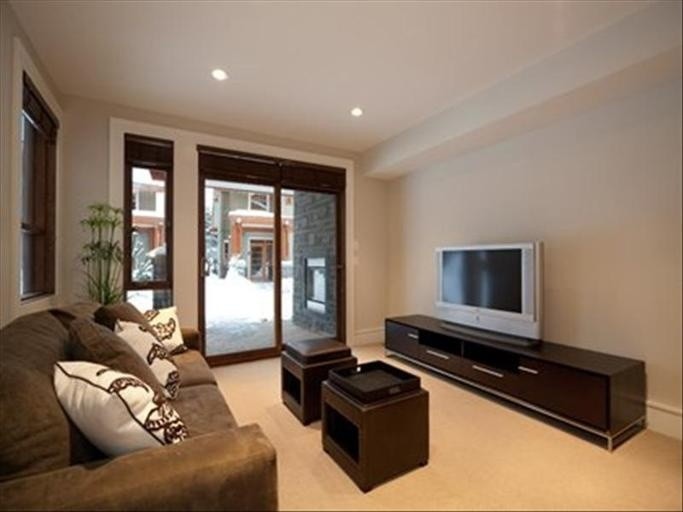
[384,313,648,454]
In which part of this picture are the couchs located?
[0,300,278,512]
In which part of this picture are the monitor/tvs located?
[434,240,545,347]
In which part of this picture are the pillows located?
[53,300,191,459]
